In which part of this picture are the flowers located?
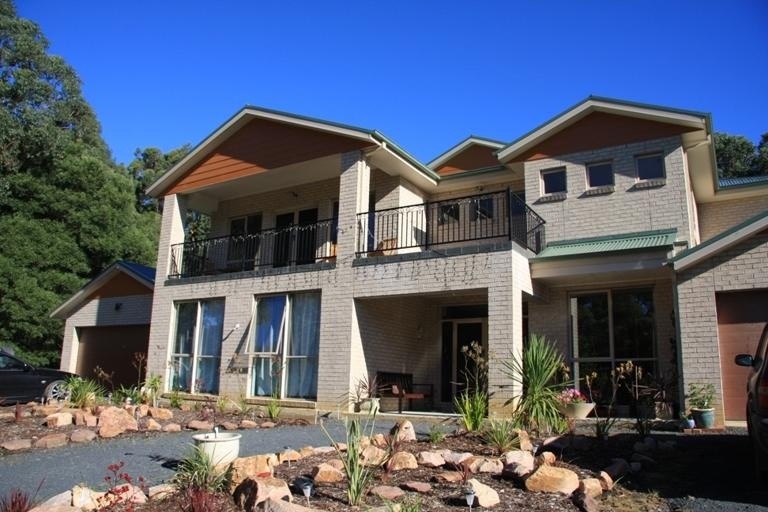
[556,389,589,407]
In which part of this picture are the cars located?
[732,321,767,462]
[173,359,192,390]
[0,348,81,407]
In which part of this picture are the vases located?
[558,402,596,419]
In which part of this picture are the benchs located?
[376,371,435,414]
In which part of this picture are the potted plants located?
[641,370,679,421]
[679,383,716,428]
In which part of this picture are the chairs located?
[367,239,396,256]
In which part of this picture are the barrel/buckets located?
[192,433,243,470]
